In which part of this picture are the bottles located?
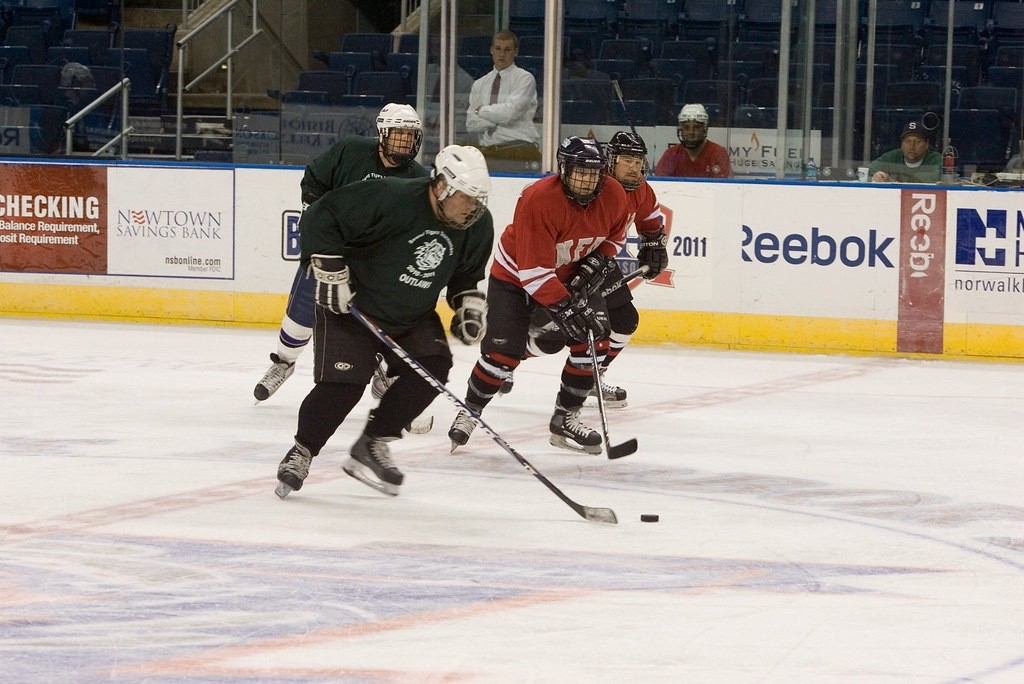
[943,138,953,174]
[805,157,817,181]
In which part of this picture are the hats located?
[900,122,930,142]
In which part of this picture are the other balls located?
[641,515,659,523]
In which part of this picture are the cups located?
[858,168,869,182]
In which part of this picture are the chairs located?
[204,0,1023,188]
[0,0,180,161]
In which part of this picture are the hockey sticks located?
[348,301,617,524]
[612,80,652,176]
[376,364,434,434]
[528,265,650,338]
[579,285,638,460]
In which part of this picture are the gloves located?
[638,246,669,279]
[310,255,357,314]
[568,253,616,297]
[451,291,487,346]
[546,297,605,345]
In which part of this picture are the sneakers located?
[448,401,482,454]
[588,378,628,409]
[274,438,312,499]
[499,371,513,394]
[549,405,602,456]
[253,353,295,407]
[370,405,434,434]
[343,434,404,496]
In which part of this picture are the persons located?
[655,104,734,178]
[423,42,474,164]
[42,63,94,156]
[1003,139,1024,173]
[274,145,493,499]
[466,31,540,172]
[450,137,631,453]
[522,131,669,406]
[867,122,942,182]
[254,103,426,406]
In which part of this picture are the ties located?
[488,72,501,134]
[427,76,440,128]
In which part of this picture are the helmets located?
[557,134,607,205]
[606,131,650,191]
[375,103,424,166]
[676,103,709,149]
[431,145,489,231]
[400,65,412,79]
[60,62,97,98]
